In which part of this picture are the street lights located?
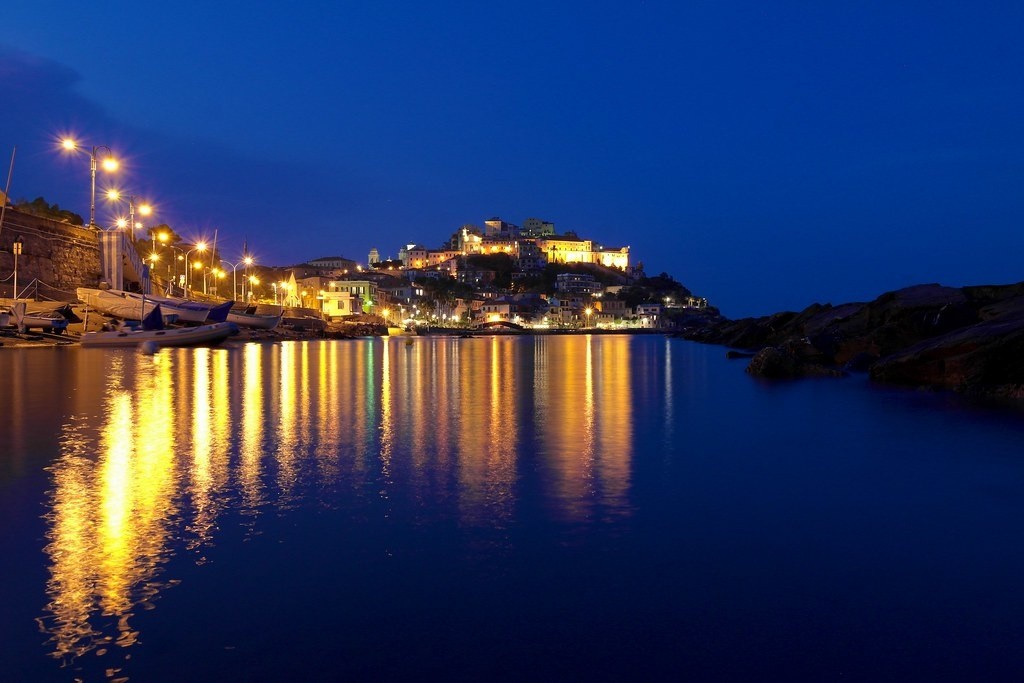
[62,137,119,231]
[134,222,169,269]
[300,291,307,306]
[272,282,278,305]
[585,308,592,327]
[279,282,293,307]
[382,310,389,325]
[161,236,259,304]
[108,189,153,242]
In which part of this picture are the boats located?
[387,327,420,337]
[78,321,239,348]
[0,304,68,336]
[281,315,328,330]
